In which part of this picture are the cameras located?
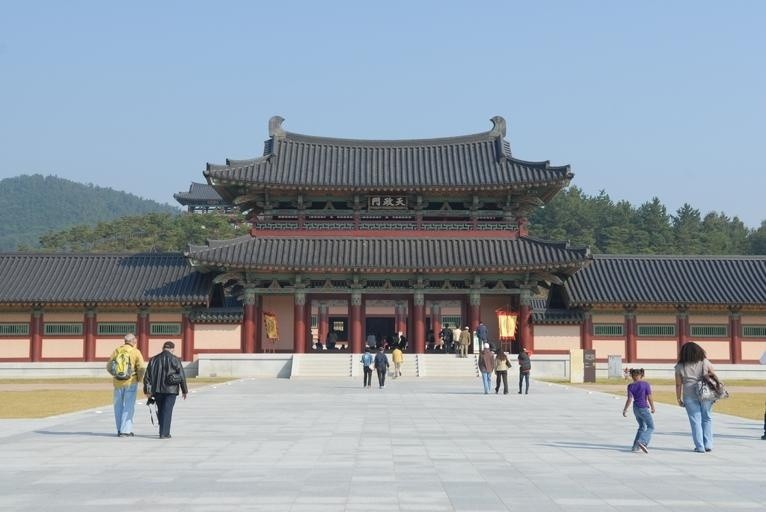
[146,397,154,405]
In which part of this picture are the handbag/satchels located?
[696,373,729,401]
[506,360,512,368]
[369,362,376,371]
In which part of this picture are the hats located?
[464,326,469,330]
[483,342,490,349]
[162,341,175,348]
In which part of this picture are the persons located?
[675,342,723,453]
[761,348,766,441]
[425,322,488,358]
[316,328,337,351]
[623,368,657,453]
[361,344,404,389]
[478,341,532,393]
[107,333,189,439]
[366,327,406,354]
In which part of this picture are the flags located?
[495,308,520,342]
[263,311,280,342]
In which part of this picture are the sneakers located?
[631,440,648,453]
[694,447,711,452]
[159,433,172,439]
[495,388,528,394]
[761,431,766,441]
[118,430,135,437]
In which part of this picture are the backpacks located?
[167,373,182,385]
[111,347,134,380]
